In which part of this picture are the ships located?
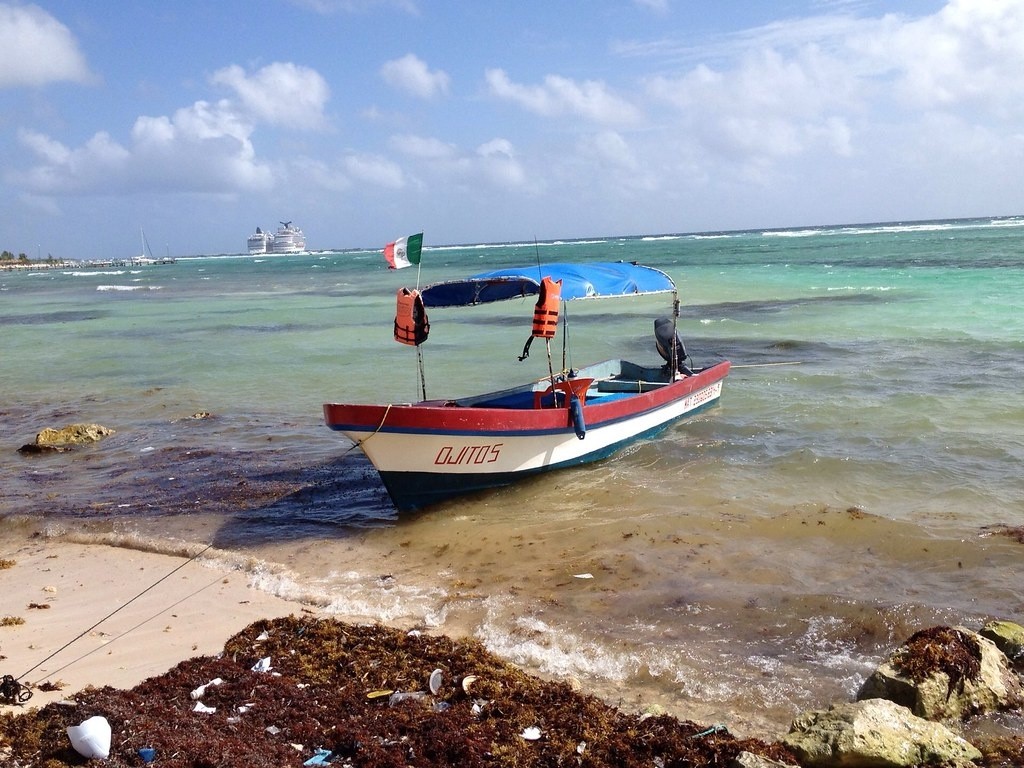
[266,221,307,255]
[247,225,272,255]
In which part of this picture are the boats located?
[2,227,179,271]
[322,233,733,510]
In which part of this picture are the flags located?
[385,233,423,269]
